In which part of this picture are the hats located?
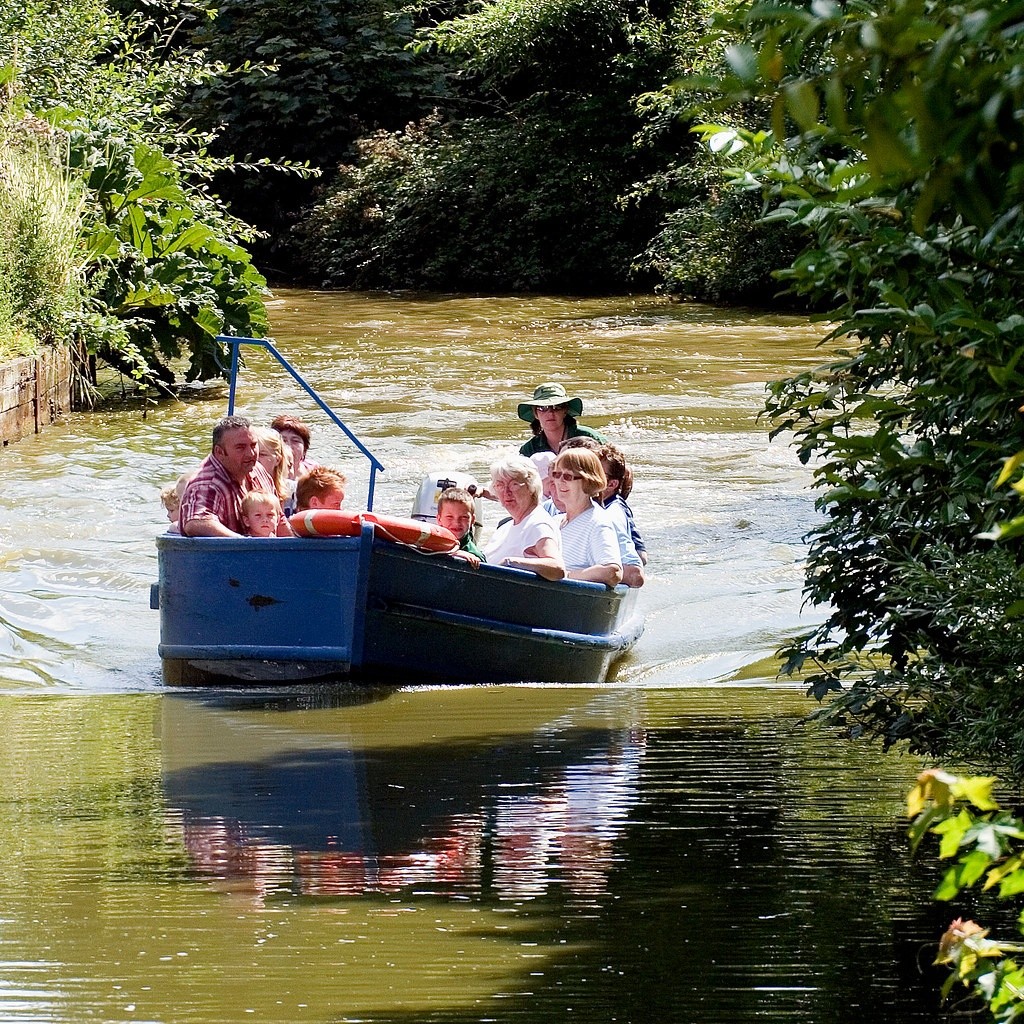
[518,383,583,422]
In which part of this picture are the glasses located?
[492,482,526,493]
[552,471,584,482]
[536,404,565,413]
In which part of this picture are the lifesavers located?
[287,509,459,554]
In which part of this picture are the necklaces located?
[563,517,570,528]
[558,431,566,448]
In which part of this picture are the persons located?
[437,487,487,570]
[483,382,648,588]
[160,416,345,537]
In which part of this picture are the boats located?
[150,336,644,687]
